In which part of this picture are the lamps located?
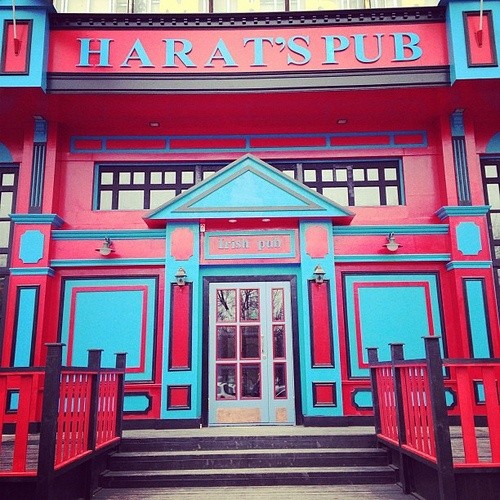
[95,237,116,256]
[313,264,325,283]
[382,232,401,252]
[175,267,187,286]
